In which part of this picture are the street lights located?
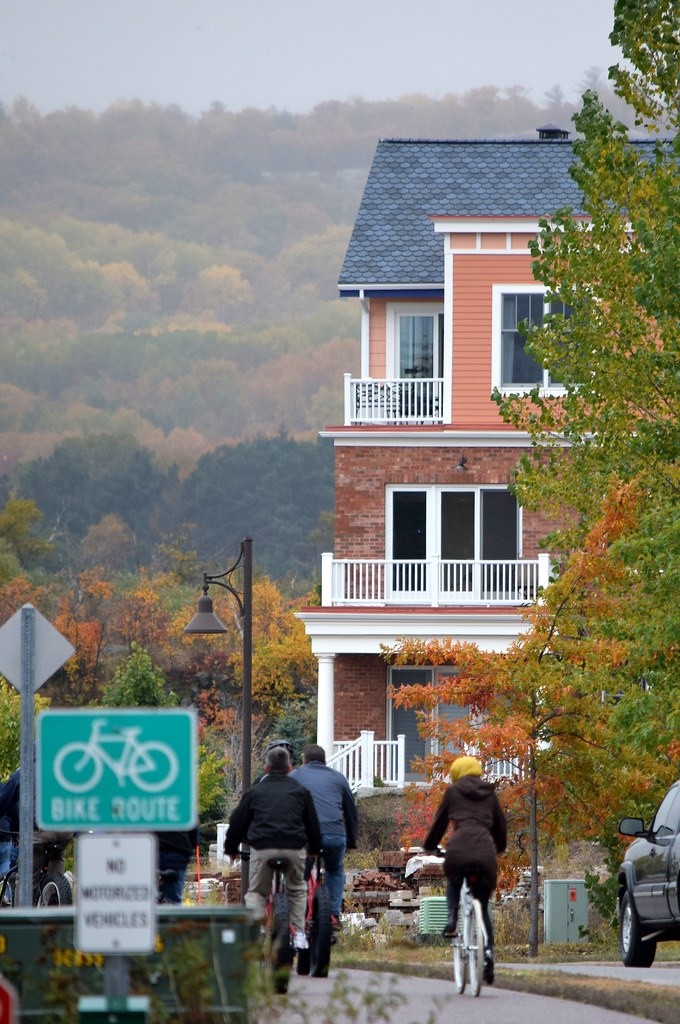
[184,535,257,912]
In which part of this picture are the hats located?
[449,757,482,782]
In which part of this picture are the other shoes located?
[331,914,344,932]
[442,914,456,937]
[484,947,495,985]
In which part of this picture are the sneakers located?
[293,931,310,950]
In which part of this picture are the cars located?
[612,773,680,970]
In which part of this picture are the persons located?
[0,761,73,881]
[158,824,200,904]
[287,745,361,929]
[1,816,15,906]
[421,756,510,986]
[250,739,297,784]
[221,744,310,953]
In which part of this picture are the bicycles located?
[227,844,295,996]
[426,846,490,999]
[294,839,360,979]
